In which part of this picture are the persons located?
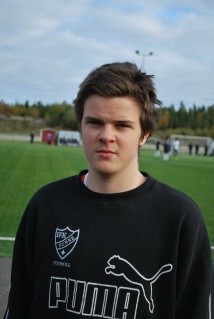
[27,130,213,163]
[2,61,214,319]
